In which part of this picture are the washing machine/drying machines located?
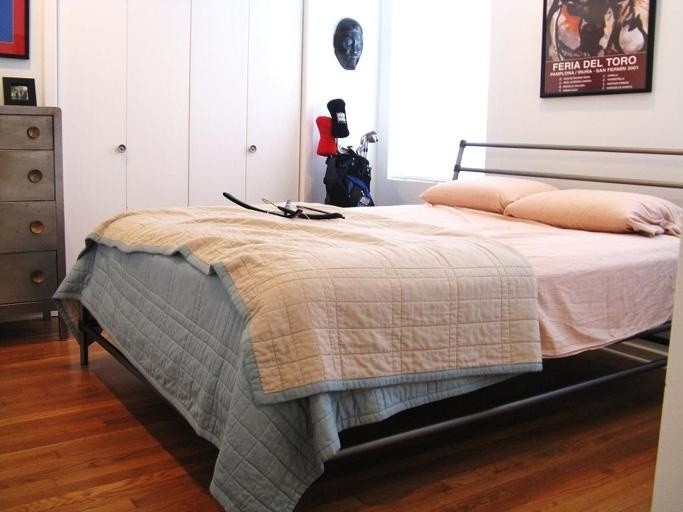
[357,132,378,159]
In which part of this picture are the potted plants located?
[1,0,31,62]
[537,0,657,100]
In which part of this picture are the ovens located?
[71,139,683,511]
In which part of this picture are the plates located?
[2,104,72,343]
[45,0,304,276]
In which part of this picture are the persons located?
[334,16,364,70]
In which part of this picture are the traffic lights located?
[420,178,682,236]
